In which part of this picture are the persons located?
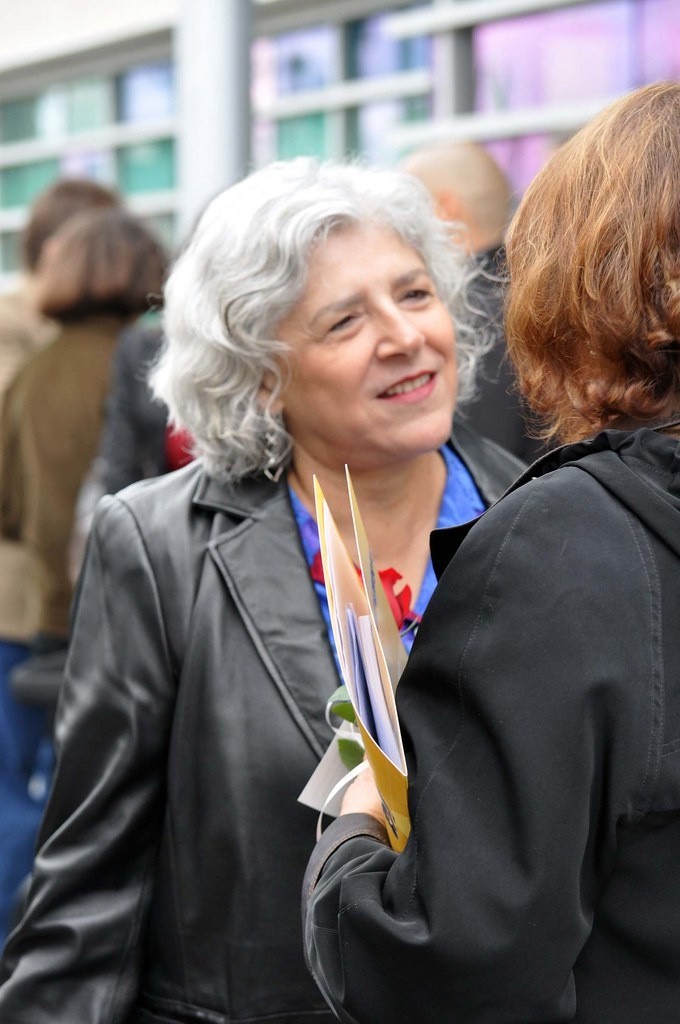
[0,205,173,659]
[0,153,534,1024]
[394,139,563,468]
[300,79,679,1024]
[0,176,143,401]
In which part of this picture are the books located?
[297,464,412,857]
